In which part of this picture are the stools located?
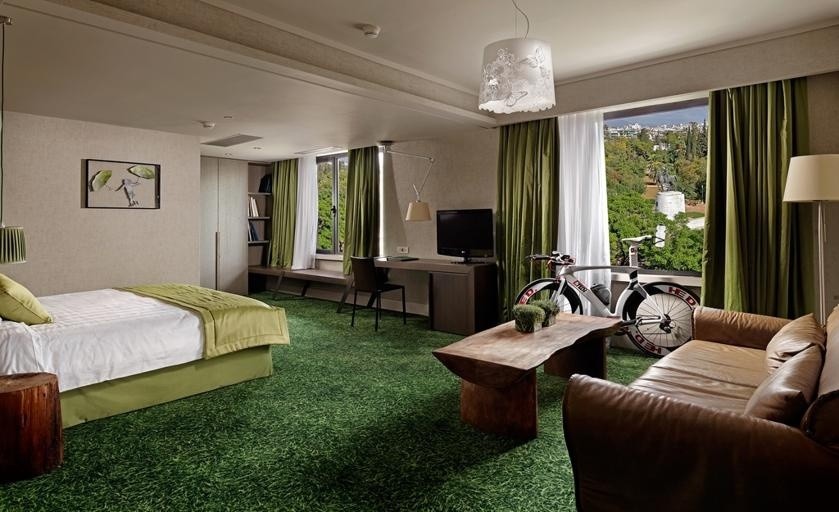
[0,372,64,479]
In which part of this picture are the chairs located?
[350,256,406,332]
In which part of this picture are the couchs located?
[563,304,839,511]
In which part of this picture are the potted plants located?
[514,305,545,333]
[532,300,560,327]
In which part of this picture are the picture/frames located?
[86,159,161,209]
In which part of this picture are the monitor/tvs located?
[437,209,494,264]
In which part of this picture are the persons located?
[114,179,140,206]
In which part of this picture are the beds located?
[0,283,290,431]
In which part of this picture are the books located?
[387,256,419,262]
[247,174,272,241]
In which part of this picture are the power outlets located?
[397,246,408,253]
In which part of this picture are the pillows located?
[743,345,823,422]
[765,313,826,376]
[0,273,54,325]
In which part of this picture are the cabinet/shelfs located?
[248,161,271,266]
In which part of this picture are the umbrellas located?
[92,170,114,193]
[128,164,155,181]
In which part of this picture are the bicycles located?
[518,235,702,357]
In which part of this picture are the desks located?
[373,257,497,336]
[248,266,353,313]
[431,310,622,437]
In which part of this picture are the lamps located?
[360,25,382,38]
[479,1,556,114]
[377,141,435,221]
[0,15,27,265]
[202,121,215,128]
[783,153,839,331]
